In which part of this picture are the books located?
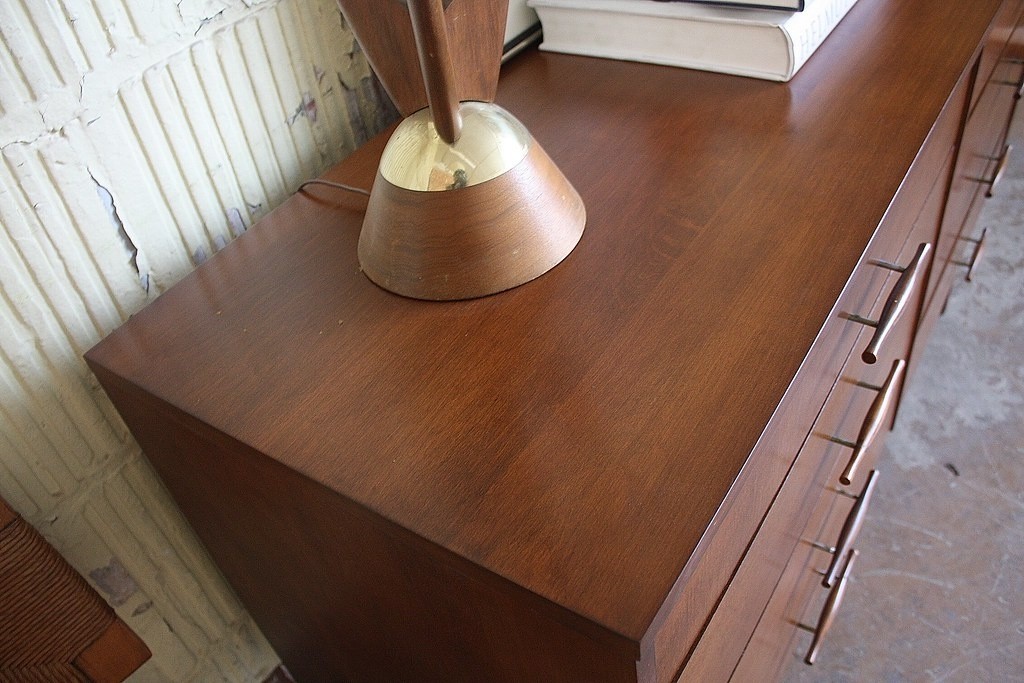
[526,0,859,83]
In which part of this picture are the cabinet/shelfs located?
[84,0,1024,683]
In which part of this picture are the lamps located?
[335,0,591,305]
